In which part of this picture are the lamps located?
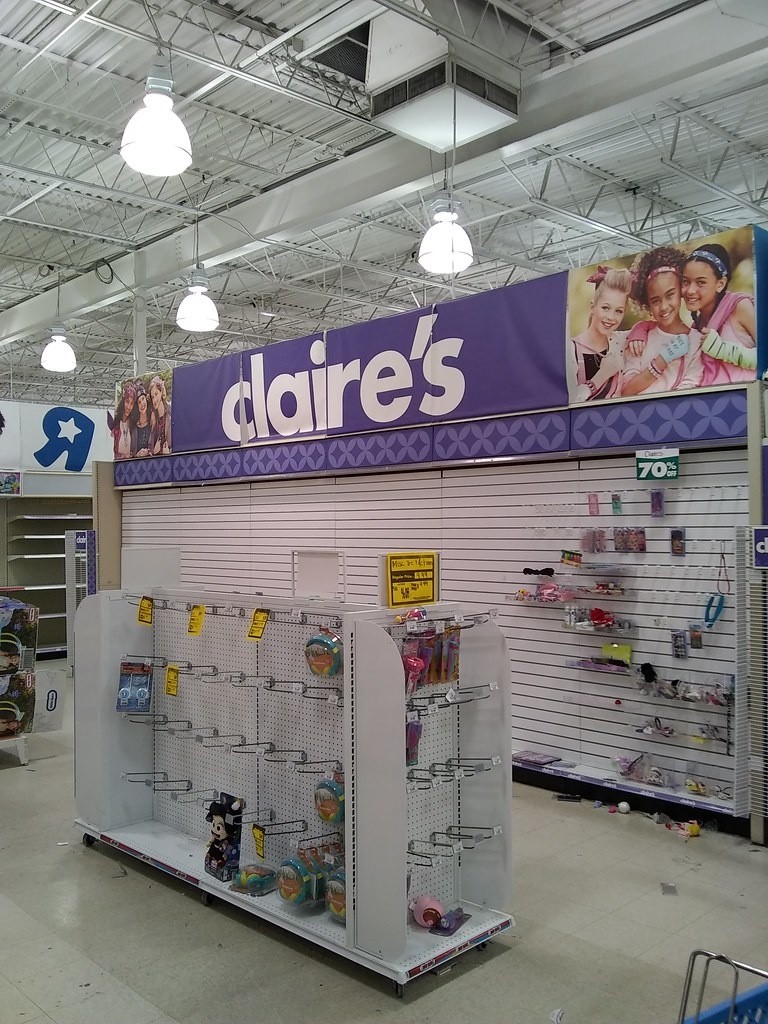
[418,31,474,275]
[40,267,77,372]
[119,37,193,177]
[175,194,220,332]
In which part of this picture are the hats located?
[136,385,147,399]
[123,382,136,399]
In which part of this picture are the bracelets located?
[585,380,598,395]
[651,359,664,374]
[646,362,661,379]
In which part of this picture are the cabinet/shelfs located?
[505,564,637,680]
[0,495,92,656]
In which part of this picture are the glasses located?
[137,389,146,396]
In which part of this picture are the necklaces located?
[122,422,128,446]
[138,418,148,425]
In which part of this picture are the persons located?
[566,244,757,403]
[0,651,20,670]
[205,802,235,868]
[0,719,21,733]
[114,375,172,460]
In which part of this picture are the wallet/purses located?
[601,643,632,666]
